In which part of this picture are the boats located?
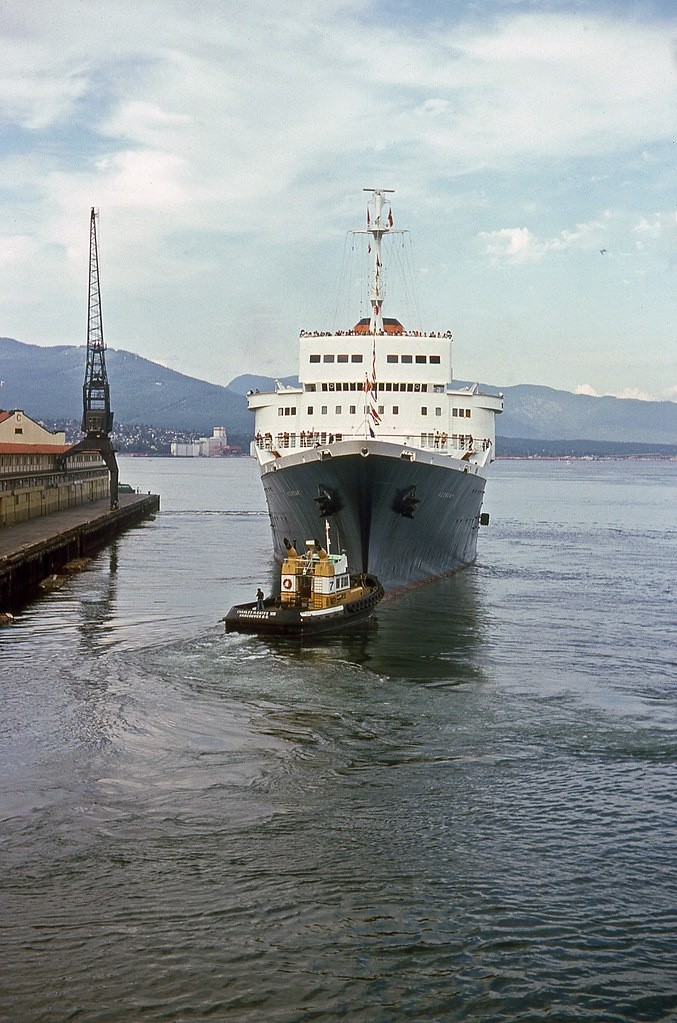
[222,520,385,638]
[244,188,504,594]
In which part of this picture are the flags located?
[376,301,384,332]
[388,208,393,226]
[368,421,375,438]
[370,403,382,421]
[364,368,377,392]
[370,413,379,425]
[368,211,370,223]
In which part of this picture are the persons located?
[312,433,319,444]
[250,385,420,394]
[483,438,492,451]
[256,588,264,611]
[279,432,288,447]
[468,434,473,451]
[255,432,272,449]
[329,433,333,444]
[304,328,451,339]
[301,430,311,447]
[435,431,446,449]
[462,436,464,450]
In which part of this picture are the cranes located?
[54,206,119,511]
